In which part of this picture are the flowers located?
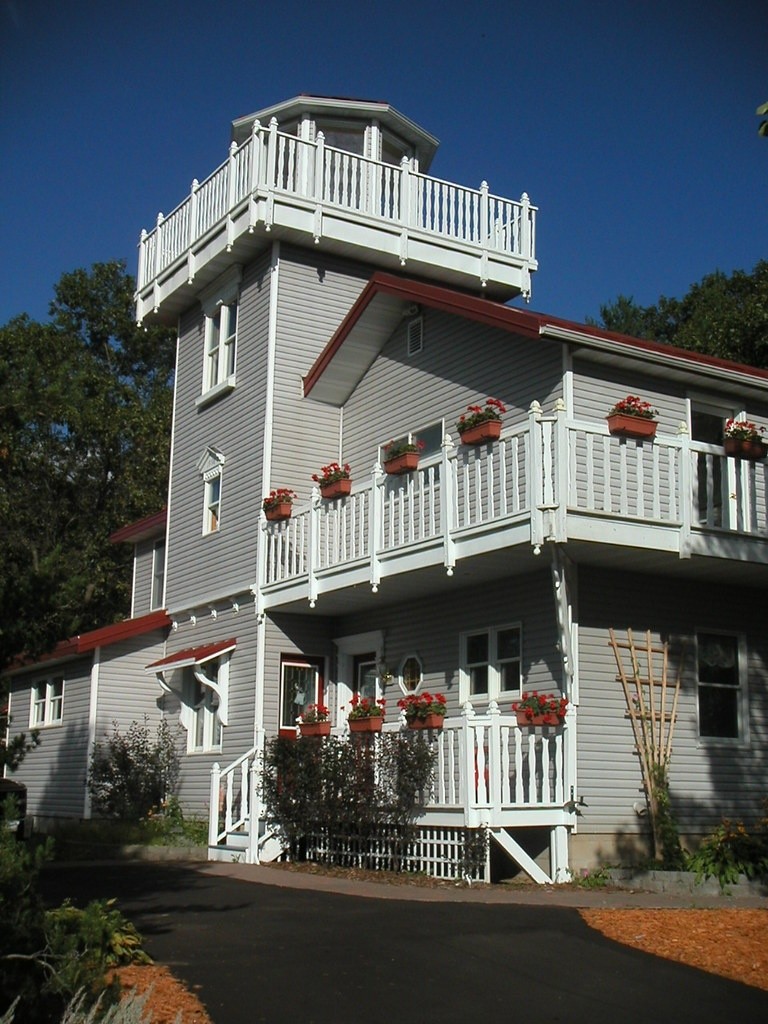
[379,439,425,457]
[610,396,660,419]
[348,692,387,719]
[312,462,352,485]
[455,399,505,431]
[300,704,330,723]
[263,488,297,510]
[511,690,569,722]
[396,692,448,716]
[723,417,768,442]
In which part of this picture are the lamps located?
[377,656,387,695]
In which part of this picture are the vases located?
[296,721,331,737]
[516,709,561,726]
[460,419,502,445]
[605,413,659,439]
[406,712,444,729]
[264,502,292,521]
[320,478,353,500]
[723,437,768,460]
[383,452,421,476]
[348,716,383,732]
